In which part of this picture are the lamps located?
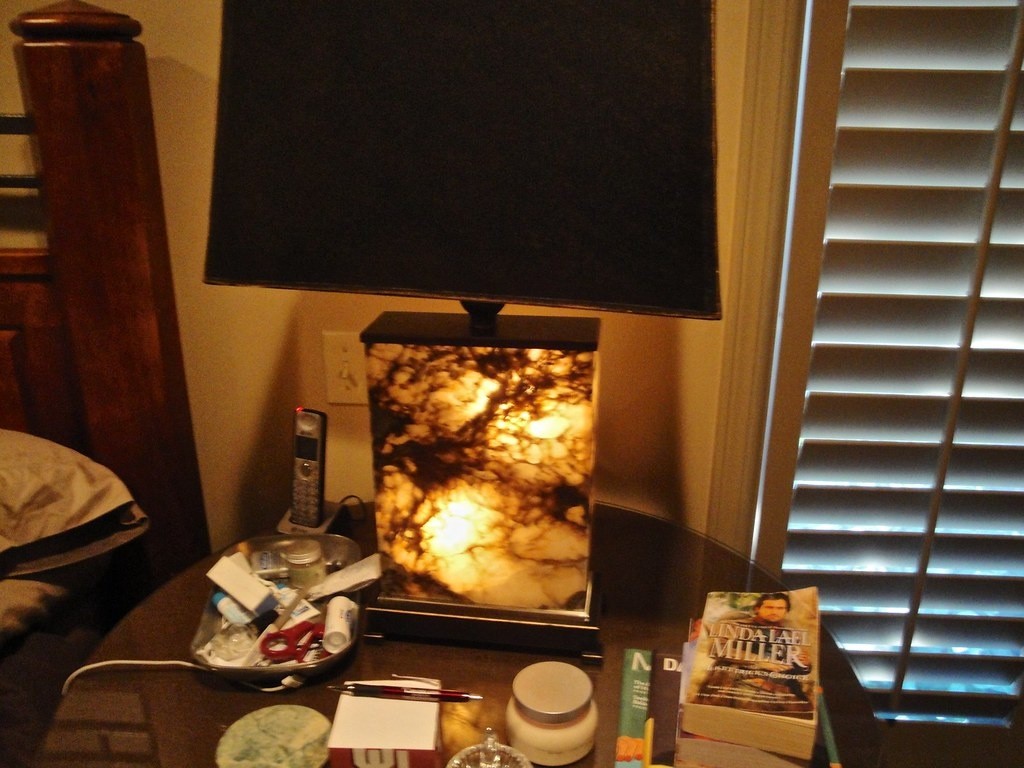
[203,0,720,662]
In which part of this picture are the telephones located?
[276,408,349,536]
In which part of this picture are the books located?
[615,586,842,768]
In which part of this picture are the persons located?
[705,593,813,717]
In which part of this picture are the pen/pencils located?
[329,683,483,704]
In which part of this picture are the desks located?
[39,499,881,767]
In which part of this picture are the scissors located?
[260,599,328,665]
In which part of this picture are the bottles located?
[323,596,354,653]
[287,540,327,598]
[211,593,254,627]
[505,661,597,766]
